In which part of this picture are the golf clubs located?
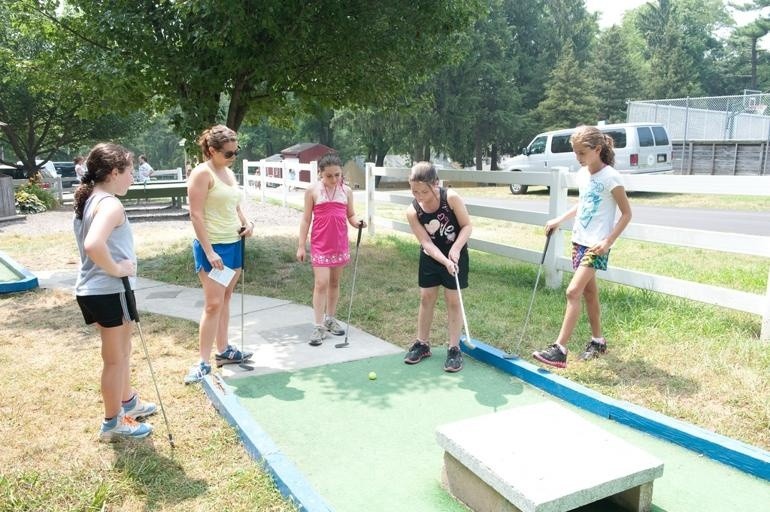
[504,228,553,359]
[240,227,253,370]
[455,270,475,350]
[335,220,363,348]
[122,276,178,448]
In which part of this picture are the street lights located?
[177,137,190,180]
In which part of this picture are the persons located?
[296,154,369,347]
[73,155,87,186]
[531,126,633,370]
[69,141,157,440]
[182,123,257,387]
[402,161,475,373]
[133,155,155,205]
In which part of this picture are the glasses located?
[217,149,239,159]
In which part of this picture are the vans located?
[53,160,79,188]
[0,163,60,195]
[501,120,676,195]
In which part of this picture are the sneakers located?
[122,396,156,418]
[404,337,432,364]
[185,361,212,385]
[532,344,568,369]
[98,407,153,440]
[215,345,253,368]
[309,325,326,346]
[444,344,464,372]
[580,337,607,362]
[325,314,345,335]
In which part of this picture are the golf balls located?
[369,372,376,380]
